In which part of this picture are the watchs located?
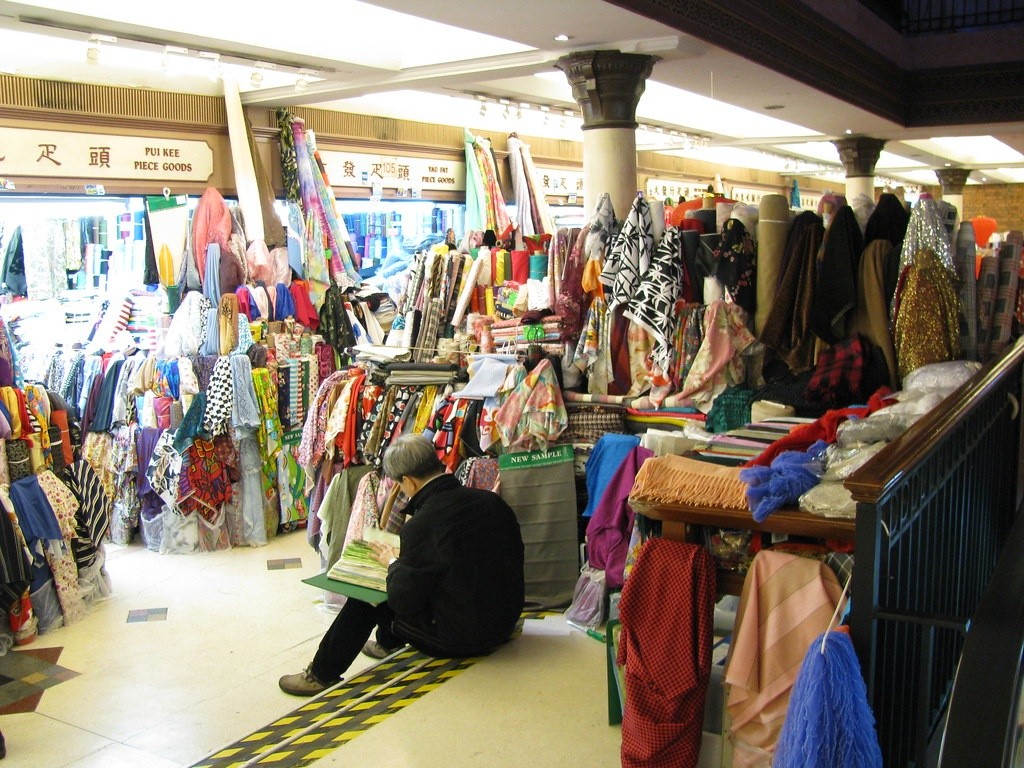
[388,557,399,567]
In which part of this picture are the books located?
[327,526,401,593]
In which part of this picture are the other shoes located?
[362,639,400,658]
[278,664,326,695]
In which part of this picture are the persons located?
[278,433,525,696]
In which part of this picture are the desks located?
[626,420,857,597]
